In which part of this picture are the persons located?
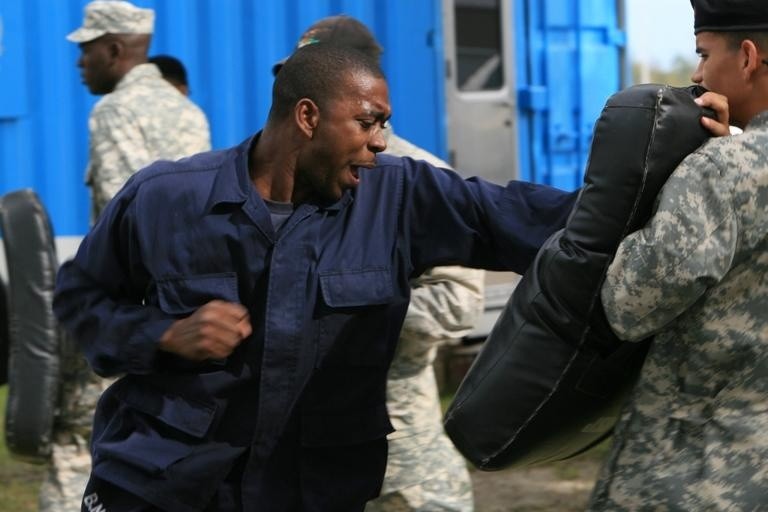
[51,41,585,511]
[268,13,483,511]
[38,2,211,512]
[143,54,188,99]
[583,0,768,512]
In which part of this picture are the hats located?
[64,1,156,44]
[269,16,386,77]
[688,1,768,35]
